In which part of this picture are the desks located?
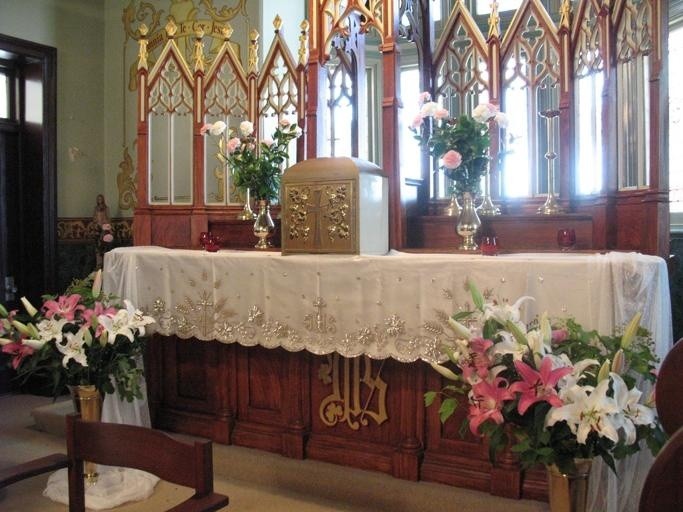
[100,244,676,511]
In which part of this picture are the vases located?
[455,190,482,253]
[547,459,590,511]
[251,199,277,249]
[64,383,106,485]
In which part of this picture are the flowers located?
[424,280,670,485]
[201,114,302,205]
[406,89,508,194]
[93,222,118,250]
[0,269,156,403]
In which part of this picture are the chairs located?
[1,412,230,511]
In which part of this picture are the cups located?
[480,236,498,256]
[557,228,577,252]
[200,232,212,250]
[205,234,221,252]
